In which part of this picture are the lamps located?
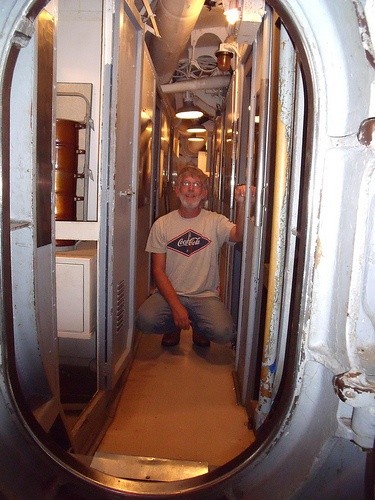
[186,118,207,133]
[175,100,204,119]
[188,133,205,142]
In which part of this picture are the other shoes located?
[192,326,210,345]
[161,329,180,346]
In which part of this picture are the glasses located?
[180,181,202,189]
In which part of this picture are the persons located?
[251,187,258,218]
[135,164,246,348]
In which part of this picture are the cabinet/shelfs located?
[55,241,99,341]
